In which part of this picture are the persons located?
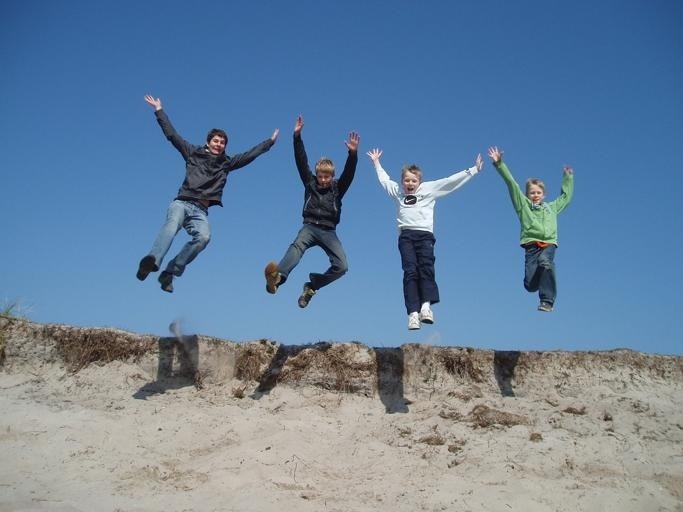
[365,148,483,329]
[486,144,572,312]
[136,93,280,293]
[263,112,361,308]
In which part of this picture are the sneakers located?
[265,260,281,293]
[298,282,317,308]
[538,301,553,312]
[408,314,421,329]
[158,272,173,292]
[136,255,155,280]
[420,308,433,324]
